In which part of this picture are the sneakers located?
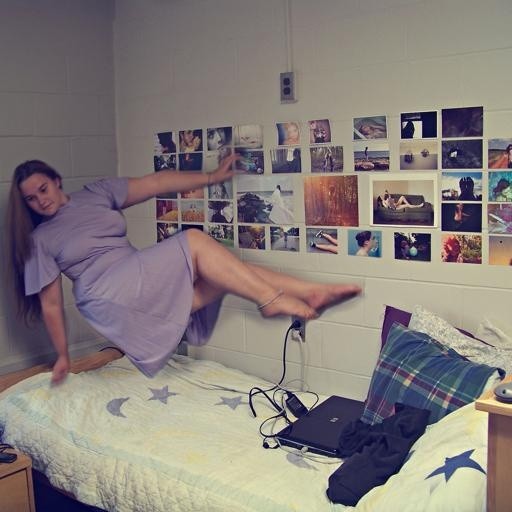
[315,229,323,237]
[310,240,317,247]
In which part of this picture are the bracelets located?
[207,173,211,187]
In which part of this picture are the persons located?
[179,129,201,152]
[207,127,227,151]
[378,190,424,210]
[356,231,379,257]
[7,152,361,385]
[157,133,175,153]
[271,184,283,208]
[358,124,386,140]
[283,122,300,144]
[309,229,338,253]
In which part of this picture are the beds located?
[0,347,489,512]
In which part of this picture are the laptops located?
[274,395,365,458]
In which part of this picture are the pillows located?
[357,303,489,511]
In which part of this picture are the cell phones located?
[0,452,18,463]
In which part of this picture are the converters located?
[286,394,308,418]
